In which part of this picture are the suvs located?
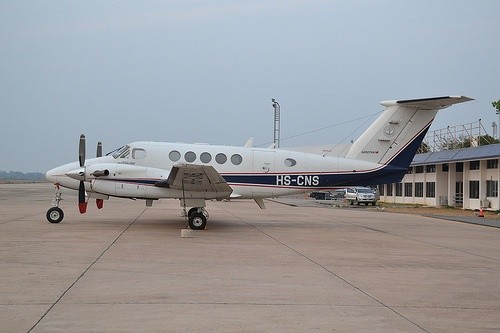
[346,187,376,206]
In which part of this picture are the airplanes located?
[45,96,477,231]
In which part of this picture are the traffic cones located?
[478,207,484,218]
